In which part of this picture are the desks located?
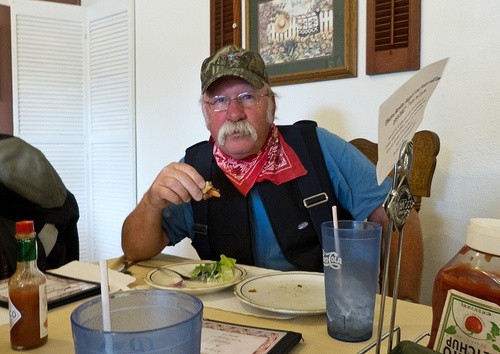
[0,253,433,354]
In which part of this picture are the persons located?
[121,44,425,304]
[0,133,80,282]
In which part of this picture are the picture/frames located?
[244,0,358,87]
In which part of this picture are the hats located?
[201,44,269,95]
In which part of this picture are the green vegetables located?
[192,254,237,282]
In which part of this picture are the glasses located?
[202,91,269,113]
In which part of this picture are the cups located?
[70,289,204,354]
[321,220,382,342]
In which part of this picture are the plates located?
[145,260,247,294]
[235,271,326,317]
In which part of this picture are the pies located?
[202,181,220,199]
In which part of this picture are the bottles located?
[427,218,500,354]
[7,220,47,350]
